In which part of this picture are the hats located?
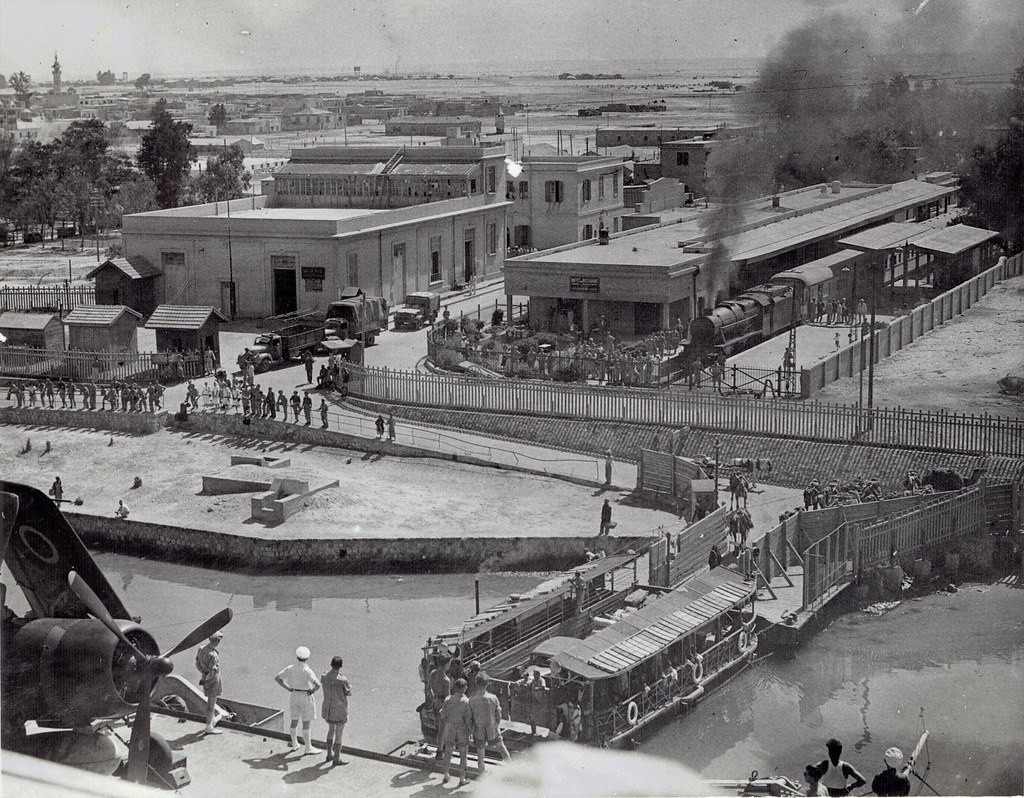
[296,646,310,660]
[885,747,904,769]
[209,630,223,642]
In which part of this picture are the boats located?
[417,548,762,750]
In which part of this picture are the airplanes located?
[0,478,234,784]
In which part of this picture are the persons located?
[419,648,545,786]
[800,298,870,350]
[514,244,537,256]
[443,305,450,321]
[7,347,396,442]
[116,499,129,518]
[52,475,63,507]
[709,544,721,570]
[803,765,830,797]
[195,629,223,734]
[816,738,867,797]
[600,499,611,534]
[604,449,613,484]
[275,646,322,754]
[459,318,727,392]
[469,273,476,296]
[321,656,352,765]
[569,570,586,616]
[871,747,910,797]
[584,546,605,562]
[804,478,838,510]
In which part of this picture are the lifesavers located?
[692,662,703,684]
[737,631,748,654]
[626,701,639,726]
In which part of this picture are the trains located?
[677,221,937,365]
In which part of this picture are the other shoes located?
[459,779,470,786]
[305,747,321,755]
[205,727,223,734]
[435,751,444,759]
[333,759,349,765]
[326,755,334,761]
[291,743,300,752]
[444,775,450,782]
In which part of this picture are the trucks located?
[238,324,327,375]
[393,291,441,330]
[322,295,389,346]
